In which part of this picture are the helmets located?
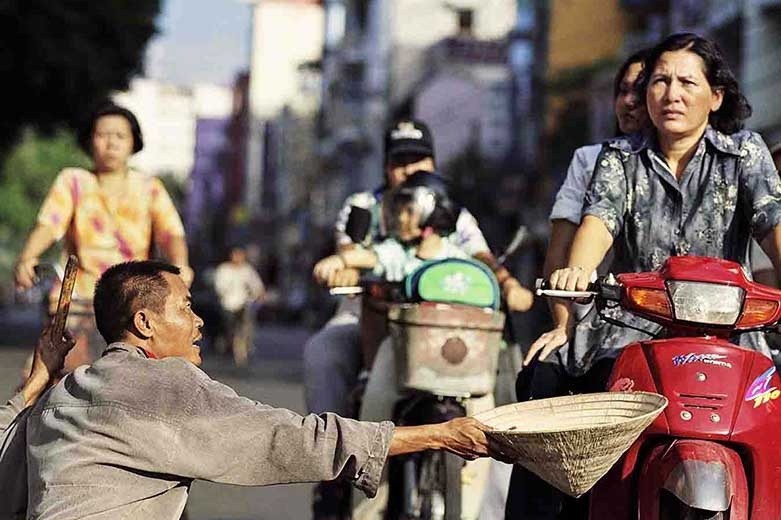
[396,170,462,236]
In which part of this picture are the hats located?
[473,392,668,499]
[385,121,434,158]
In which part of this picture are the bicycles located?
[26,263,114,391]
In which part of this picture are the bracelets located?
[336,254,347,269]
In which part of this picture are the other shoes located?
[307,481,350,517]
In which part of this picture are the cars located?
[12,270,55,305]
[190,281,340,327]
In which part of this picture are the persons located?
[26,259,517,520]
[313,170,492,485]
[505,33,781,520]
[213,243,266,363]
[14,102,193,404]
[0,319,76,520]
[302,118,533,520]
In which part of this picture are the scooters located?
[535,256,781,520]
[323,272,514,520]
